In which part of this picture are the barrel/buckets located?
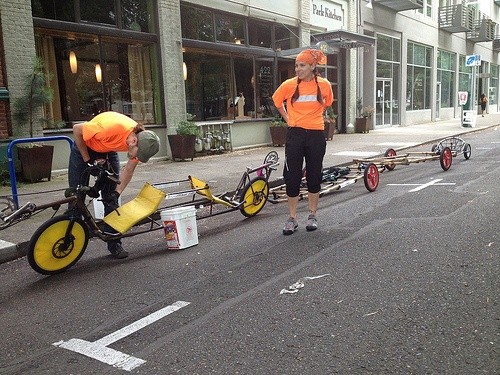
[160,205,198,250]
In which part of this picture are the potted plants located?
[356,97,373,133]
[268,120,288,144]
[168,114,200,160]
[323,107,337,141]
[13,55,64,182]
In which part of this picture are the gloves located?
[107,190,121,209]
[83,160,102,176]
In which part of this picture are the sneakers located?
[283,216,299,235]
[108,240,128,259]
[306,214,318,231]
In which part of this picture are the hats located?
[137,130,161,163]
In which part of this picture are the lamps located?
[70,50,78,73]
[95,64,102,82]
[183,62,188,80]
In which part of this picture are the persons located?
[480,94,488,117]
[68,111,160,259]
[272,49,333,235]
